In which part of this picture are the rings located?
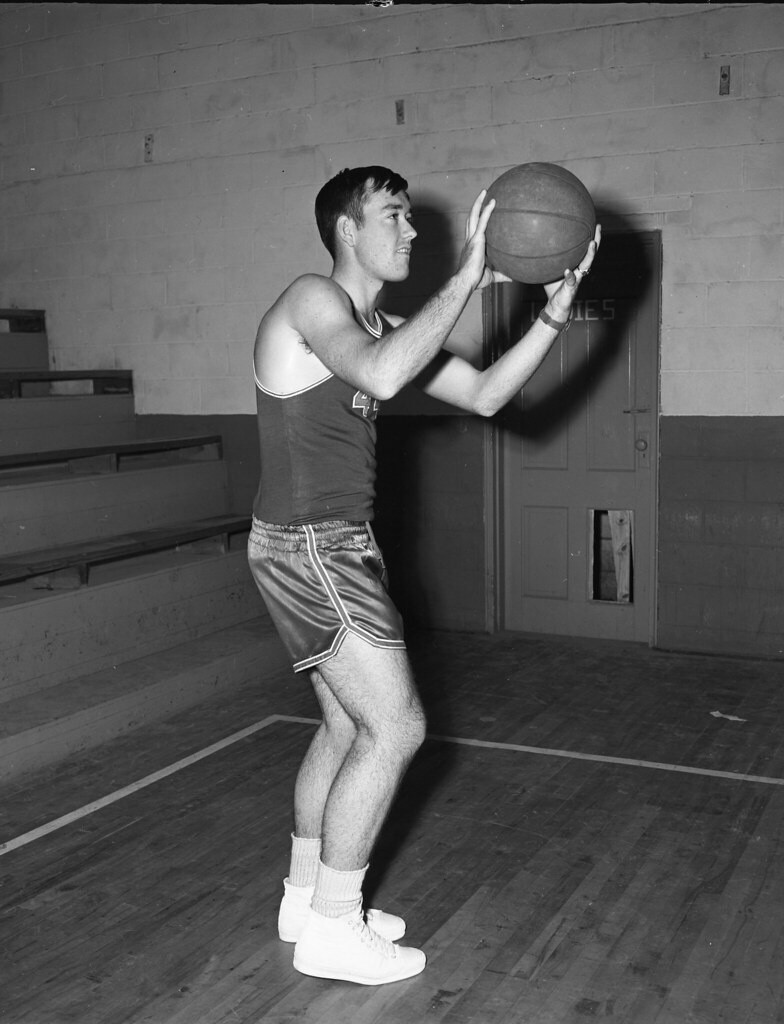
[577,266,591,276]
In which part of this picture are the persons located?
[246,165,604,987]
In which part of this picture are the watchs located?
[536,307,572,334]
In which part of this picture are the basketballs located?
[477,161,598,285]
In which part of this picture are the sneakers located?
[277,877,406,942]
[293,900,426,985]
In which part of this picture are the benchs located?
[0,512,258,587]
[0,307,45,332]
[0,433,224,485]
[0,369,135,400]
